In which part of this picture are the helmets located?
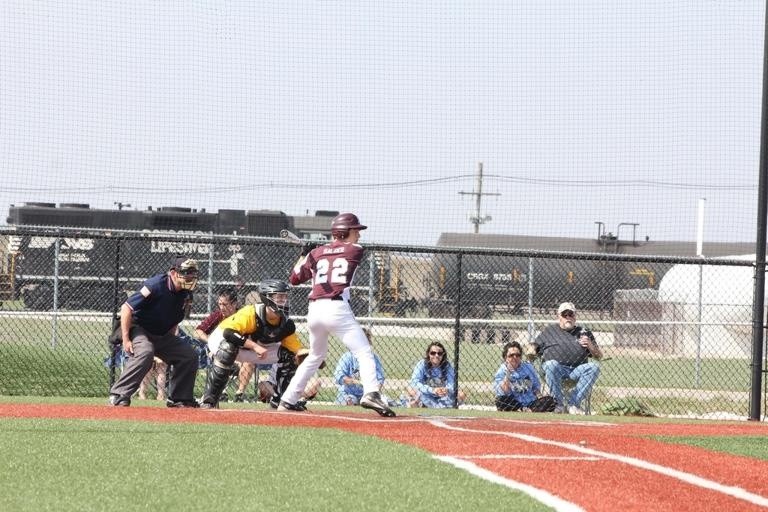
[258,279,290,314]
[330,213,366,240]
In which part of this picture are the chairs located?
[105,325,206,399]
[527,354,612,415]
[204,345,272,403]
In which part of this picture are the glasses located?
[507,352,521,359]
[560,312,574,318]
[429,351,444,356]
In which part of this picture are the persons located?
[104,254,326,412]
[525,301,602,416]
[274,212,397,419]
[469,304,496,343]
[492,341,556,413]
[399,342,465,408]
[333,328,395,407]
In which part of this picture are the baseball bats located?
[281,230,306,247]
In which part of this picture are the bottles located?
[399,390,406,408]
[579,326,589,338]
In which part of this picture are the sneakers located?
[166,397,199,408]
[110,394,131,407]
[200,396,218,410]
[359,392,396,417]
[271,396,311,413]
[568,406,596,415]
[553,405,565,414]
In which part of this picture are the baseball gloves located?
[296,349,326,369]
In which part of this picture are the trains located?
[1,200,768,329]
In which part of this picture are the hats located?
[169,257,199,271]
[558,302,577,313]
